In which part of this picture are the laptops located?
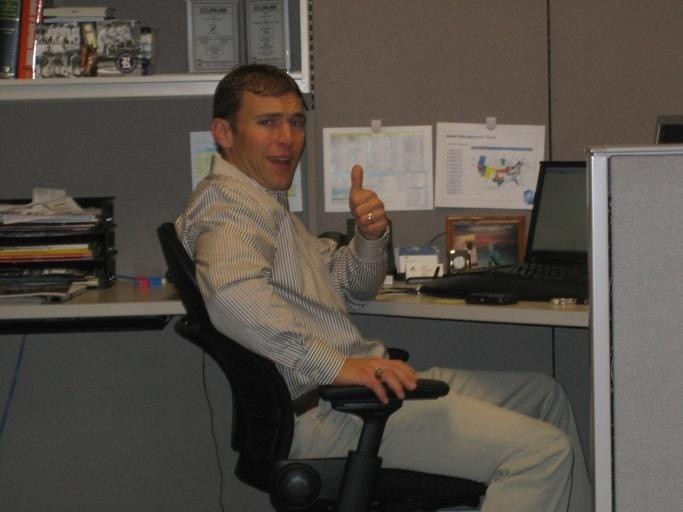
[419,160,588,301]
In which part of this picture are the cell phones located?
[465,292,519,305]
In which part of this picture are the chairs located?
[158,223,486,512]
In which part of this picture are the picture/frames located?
[446,214,527,272]
[187,1,239,74]
[245,0,291,73]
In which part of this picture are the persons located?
[36,21,152,78]
[173,61,595,512]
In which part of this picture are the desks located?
[0,277,591,327]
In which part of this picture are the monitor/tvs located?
[653,114,683,144]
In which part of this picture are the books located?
[1,0,21,78]
[16,0,44,79]
[0,205,105,301]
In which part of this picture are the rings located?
[374,367,384,381]
[368,213,375,222]
